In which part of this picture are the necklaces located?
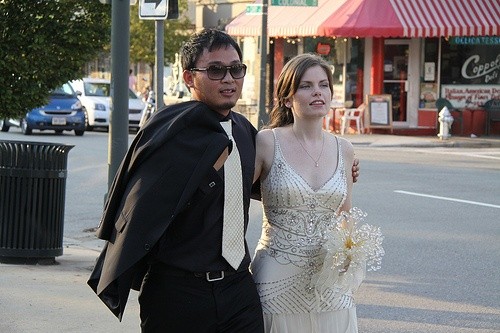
[292,124,324,167]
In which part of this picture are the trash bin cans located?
[0,138,76,264]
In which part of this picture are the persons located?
[137,29,359,333]
[129,69,137,90]
[251,54,385,333]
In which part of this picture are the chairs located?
[435,97,500,137]
[319,101,367,135]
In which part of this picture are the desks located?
[463,106,484,137]
[330,102,346,134]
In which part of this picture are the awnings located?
[226,0,500,38]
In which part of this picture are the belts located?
[148,264,248,281]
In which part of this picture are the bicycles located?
[138,87,168,127]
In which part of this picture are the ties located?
[219,119,246,270]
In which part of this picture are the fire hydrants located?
[436,106,454,140]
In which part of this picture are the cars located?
[63,76,145,133]
[0,81,85,136]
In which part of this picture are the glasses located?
[188,64,247,80]
